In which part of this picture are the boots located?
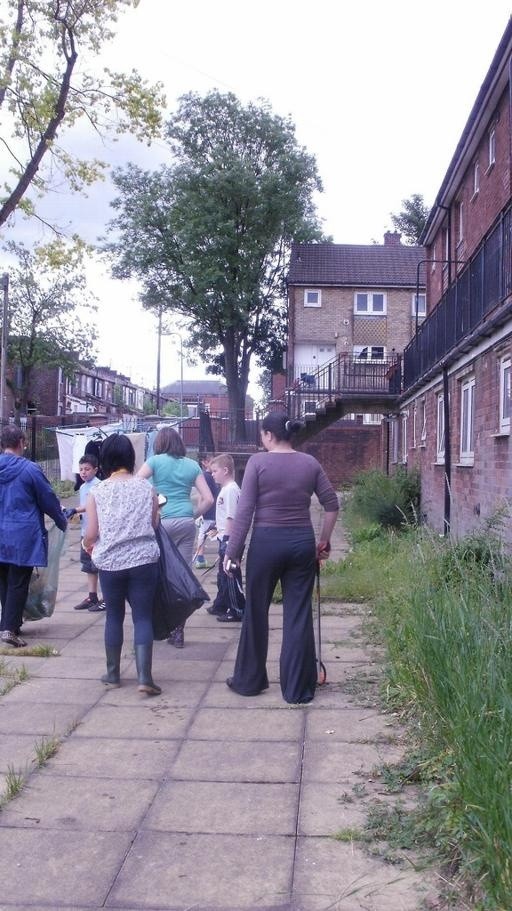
[134,643,162,695]
[100,644,123,689]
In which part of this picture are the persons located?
[80,433,163,695]
[206,454,249,622]
[66,454,109,612]
[195,456,217,569]
[0,424,68,648]
[223,411,339,705]
[136,427,214,648]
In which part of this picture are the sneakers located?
[73,596,99,610]
[206,605,227,616]
[88,598,107,612]
[226,676,260,696]
[195,559,208,569]
[1,629,28,647]
[217,607,242,623]
[167,625,185,648]
